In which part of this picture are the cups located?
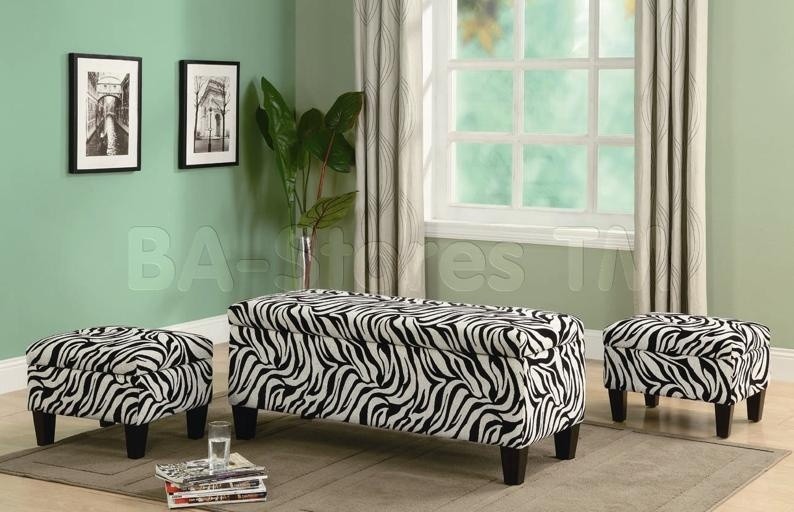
[207,420,232,470]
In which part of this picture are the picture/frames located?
[69,53,142,174]
[178,59,241,170]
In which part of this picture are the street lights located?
[208,106,213,153]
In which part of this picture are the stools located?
[602,312,771,438]
[27,326,212,459]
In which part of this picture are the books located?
[154,450,265,510]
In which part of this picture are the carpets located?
[1,390,792,511]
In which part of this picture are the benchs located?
[226,289,585,485]
